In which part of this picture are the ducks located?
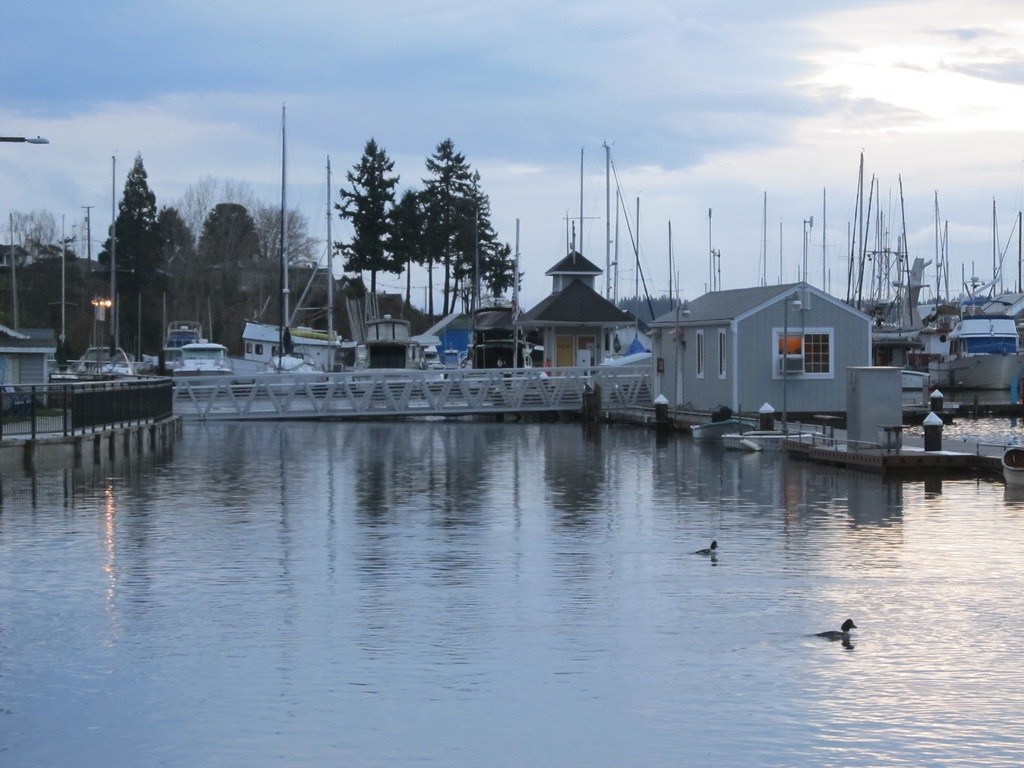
[817,619,857,637]
[696,541,718,555]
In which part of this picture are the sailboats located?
[7,98,1023,425]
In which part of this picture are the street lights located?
[0,137,52,145]
[90,294,111,376]
[783,294,802,438]
[673,305,691,420]
